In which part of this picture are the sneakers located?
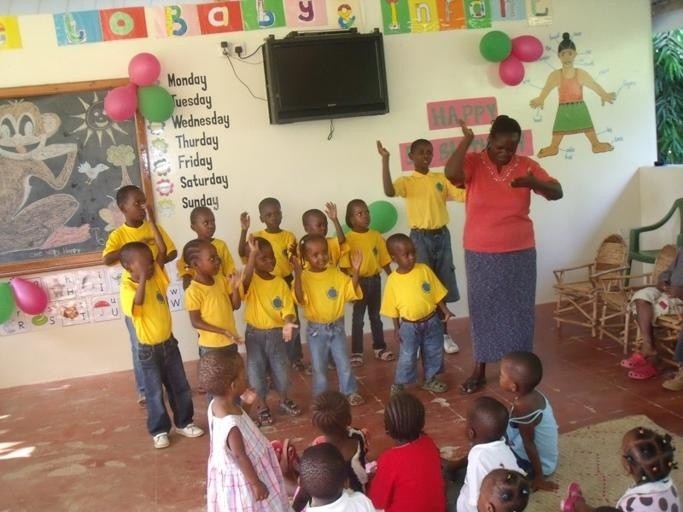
[442,334,459,353]
[662,365,683,393]
[152,433,171,450]
[175,423,204,439]
[346,393,366,406]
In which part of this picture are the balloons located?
[136,83,175,122]
[512,35,544,61]
[10,277,47,315]
[479,29,512,63]
[128,52,161,86]
[104,83,138,122]
[499,56,525,86]
[0,282,16,323]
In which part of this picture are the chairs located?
[551,230,682,372]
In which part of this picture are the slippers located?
[456,378,488,395]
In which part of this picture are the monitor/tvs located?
[262,30,392,123]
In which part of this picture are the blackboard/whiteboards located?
[0,78,157,277]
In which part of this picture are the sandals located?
[374,348,394,361]
[620,352,660,381]
[350,353,365,368]
[279,399,303,417]
[390,385,405,396]
[421,379,447,393]
[255,406,274,425]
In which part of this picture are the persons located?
[530,30,618,159]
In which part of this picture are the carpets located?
[440,408,683,512]
